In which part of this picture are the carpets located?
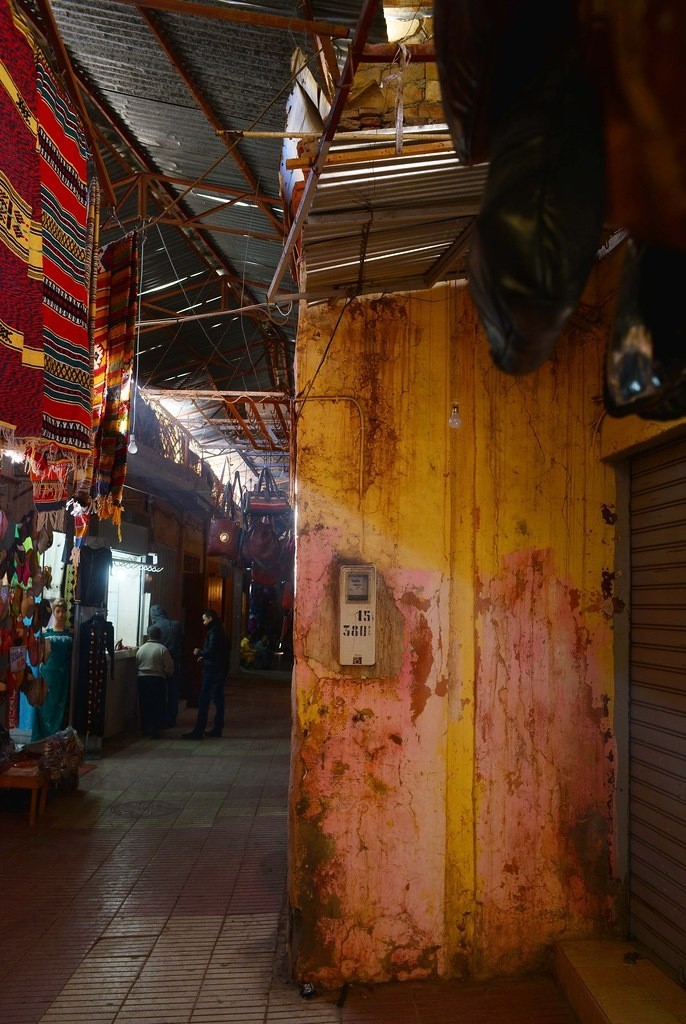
[78,764,98,778]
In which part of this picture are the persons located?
[181,606,233,740]
[36,598,115,755]
[135,604,187,742]
[240,629,276,671]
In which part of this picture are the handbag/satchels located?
[166,637,184,657]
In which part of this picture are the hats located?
[245,634,254,640]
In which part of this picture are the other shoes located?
[160,722,177,729]
[205,728,223,737]
[181,730,204,738]
[151,729,160,738]
[141,728,149,736]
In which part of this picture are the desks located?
[0,763,50,825]
